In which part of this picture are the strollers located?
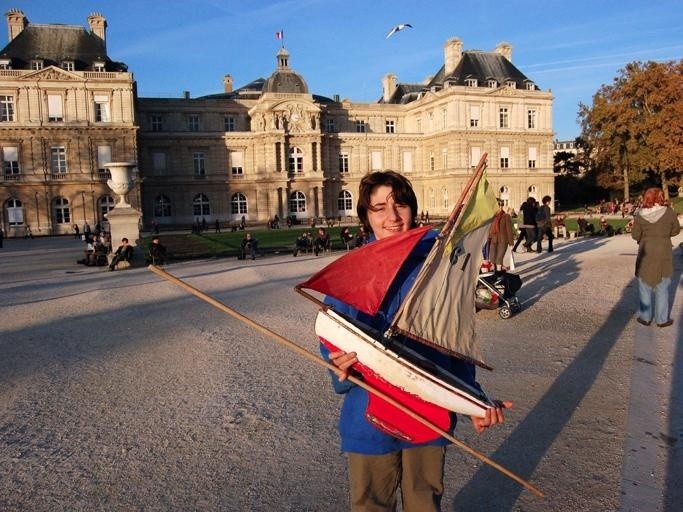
[473,260,526,318]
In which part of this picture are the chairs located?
[600,220,615,236]
[240,239,258,260]
[293,235,370,254]
[147,244,170,265]
[576,223,593,238]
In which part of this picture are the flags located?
[275,32,282,39]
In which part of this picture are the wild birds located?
[386,22,412,39]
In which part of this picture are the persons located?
[320,170,512,512]
[523,197,539,252]
[577,187,680,328]
[512,201,527,253]
[26,225,35,239]
[426,211,428,218]
[0,228,3,248]
[421,211,424,219]
[536,195,554,252]
[488,197,514,264]
[553,215,566,226]
[73,213,370,272]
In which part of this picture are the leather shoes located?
[637,317,650,325]
[657,320,673,327]
